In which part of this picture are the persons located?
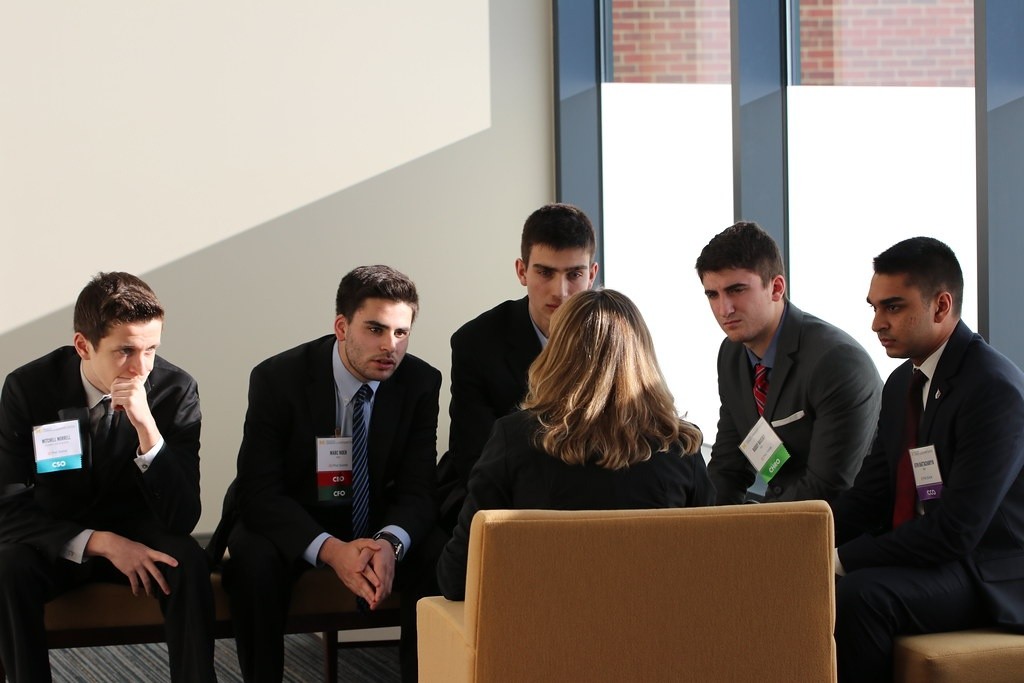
[218,261,444,683]
[433,287,718,602]
[694,220,886,541]
[434,197,605,534]
[0,272,220,683]
[822,235,1023,682]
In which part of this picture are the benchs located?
[0,566,400,683]
[894,627,1024,683]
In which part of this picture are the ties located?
[754,364,770,417]
[92,400,113,534]
[350,384,373,612]
[893,369,929,526]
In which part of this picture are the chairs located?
[417,501,837,683]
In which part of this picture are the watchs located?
[371,531,404,566]
